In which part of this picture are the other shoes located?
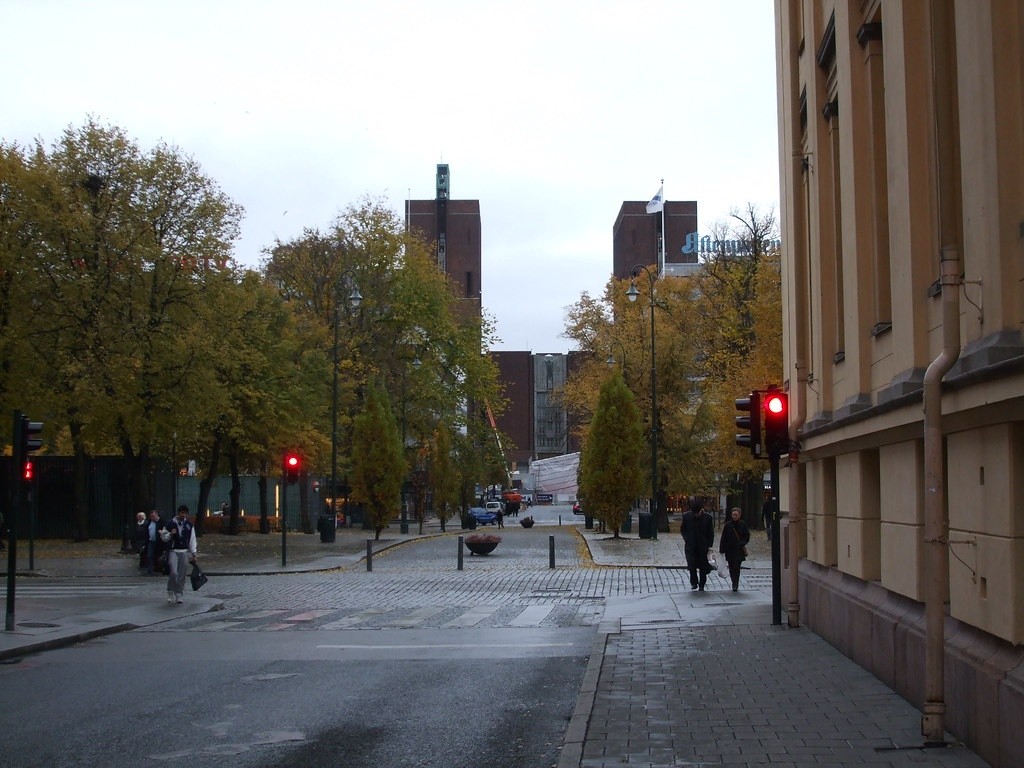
[733,585,738,591]
[699,587,704,591]
[691,585,697,589]
[176,593,183,603]
[167,592,173,602]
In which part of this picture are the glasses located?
[732,514,739,517]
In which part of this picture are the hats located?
[691,502,703,512]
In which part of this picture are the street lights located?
[623,262,660,540]
[328,268,362,544]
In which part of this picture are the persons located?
[132,509,167,577]
[761,495,772,541]
[491,489,496,499]
[680,502,715,591]
[159,506,197,604]
[491,510,504,529]
[527,497,532,508]
[221,501,231,516]
[720,506,750,591]
[505,501,520,517]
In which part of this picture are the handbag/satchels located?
[708,550,718,570]
[743,548,748,557]
[188,559,208,591]
[716,553,728,578]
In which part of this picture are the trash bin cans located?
[638,512,653,539]
[320,515,335,543]
[585,516,593,529]
[259,518,270,534]
[621,514,632,533]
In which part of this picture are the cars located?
[573,501,584,515]
[468,506,498,526]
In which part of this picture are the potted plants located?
[519,516,535,529]
[466,533,502,557]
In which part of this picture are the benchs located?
[222,517,249,534]
[259,518,291,533]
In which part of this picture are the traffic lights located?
[286,454,300,482]
[733,392,762,455]
[765,390,789,454]
[22,458,34,482]
[21,416,44,456]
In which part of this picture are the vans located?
[485,502,505,517]
